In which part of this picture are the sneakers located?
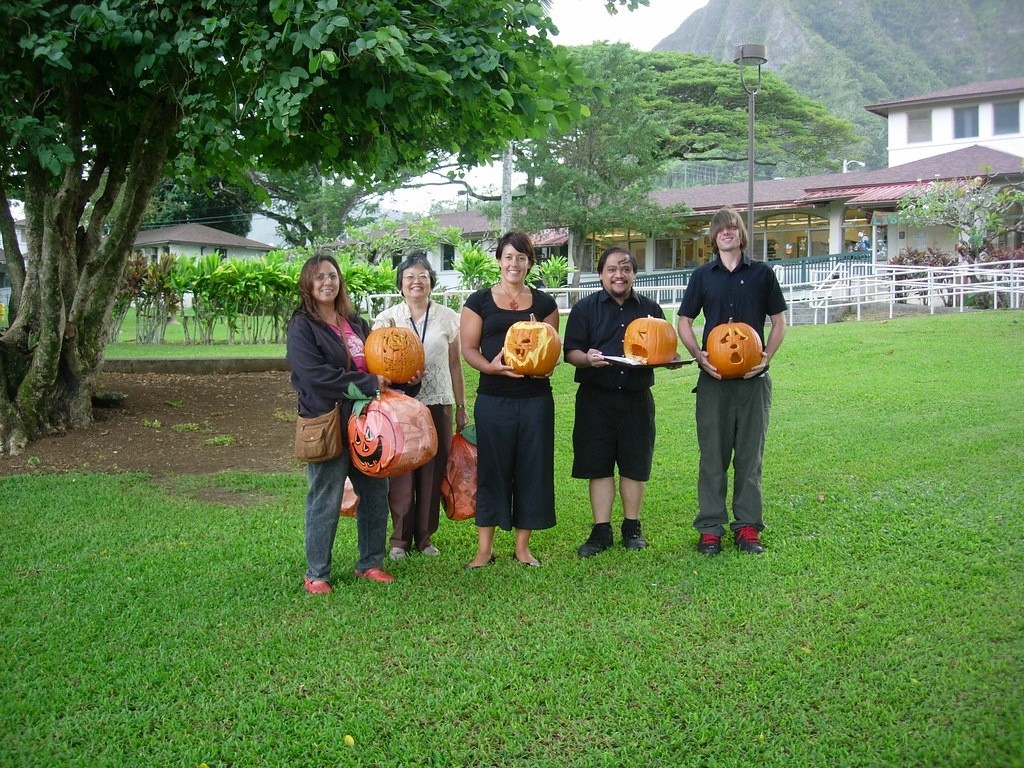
[577,525,613,558]
[697,534,722,555]
[621,525,649,548]
[734,526,763,552]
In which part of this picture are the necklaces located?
[499,283,525,309]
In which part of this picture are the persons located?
[287,253,430,597]
[370,250,469,559]
[677,204,788,557]
[460,230,559,570]
[564,247,681,559]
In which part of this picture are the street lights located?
[842,159,864,173]
[734,43,767,261]
[457,188,468,211]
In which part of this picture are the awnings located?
[489,227,571,252]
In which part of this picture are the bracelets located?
[457,405,466,408]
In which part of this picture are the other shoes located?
[422,546,440,556]
[354,567,394,583]
[389,547,406,560]
[513,552,541,567]
[466,555,495,569]
[305,576,332,594]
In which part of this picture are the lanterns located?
[503,321,561,377]
[623,318,678,364]
[365,327,425,384]
[707,322,762,378]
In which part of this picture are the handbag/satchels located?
[441,433,477,520]
[348,385,439,479]
[296,403,343,463]
[340,476,359,517]
